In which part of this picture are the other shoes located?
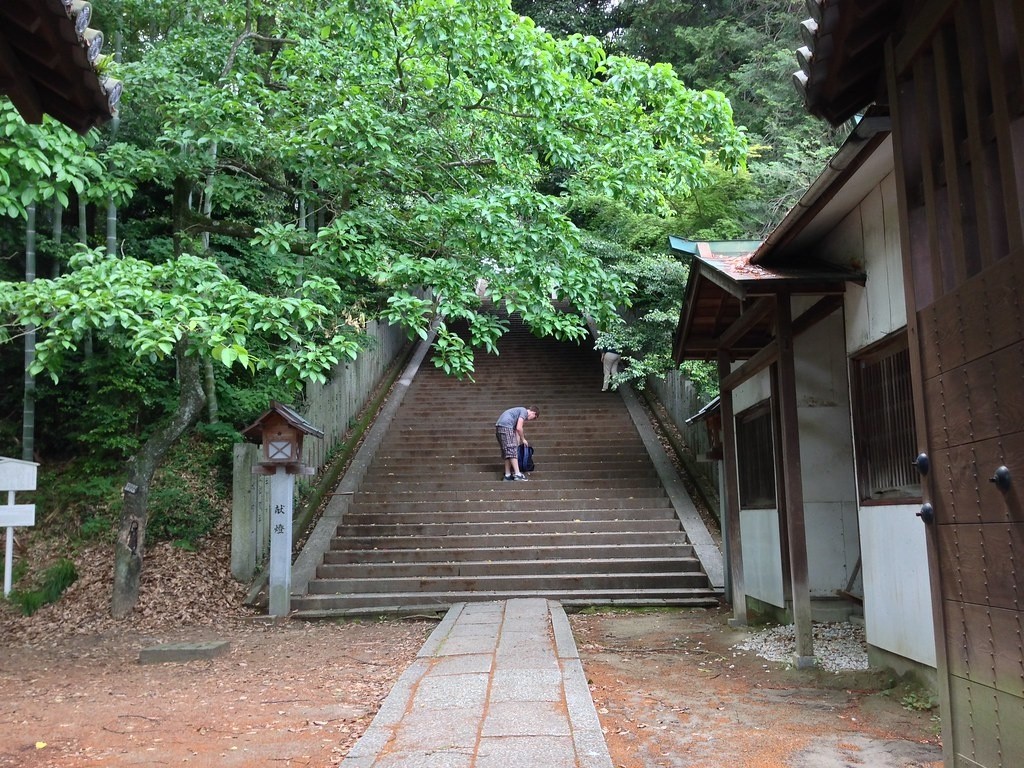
[602,388,606,391]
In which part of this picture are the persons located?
[494,405,540,482]
[600,333,621,392]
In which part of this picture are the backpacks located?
[518,443,535,472]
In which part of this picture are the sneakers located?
[513,473,528,481]
[502,474,513,481]
